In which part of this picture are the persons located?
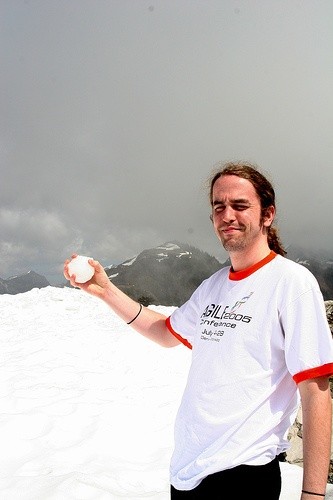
[64,165,333,500]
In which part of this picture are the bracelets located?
[126,302,142,325]
[301,490,326,496]
